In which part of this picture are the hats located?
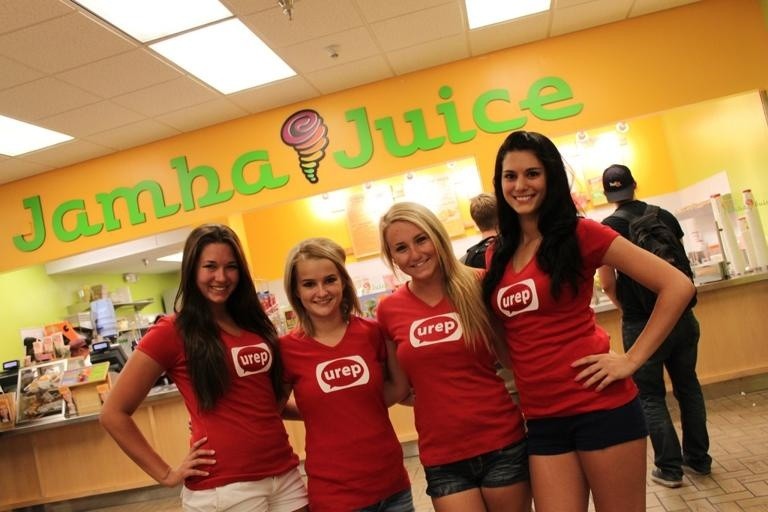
[602,164,634,203]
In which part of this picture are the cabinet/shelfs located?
[14,353,92,424]
[106,298,160,351]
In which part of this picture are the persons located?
[99,223,306,511]
[481,131,696,511]
[66,337,93,367]
[376,201,531,512]
[188,237,416,512]
[597,164,713,488]
[460,196,520,406]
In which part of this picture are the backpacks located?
[612,205,698,315]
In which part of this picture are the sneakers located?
[651,468,684,488]
[681,461,711,475]
